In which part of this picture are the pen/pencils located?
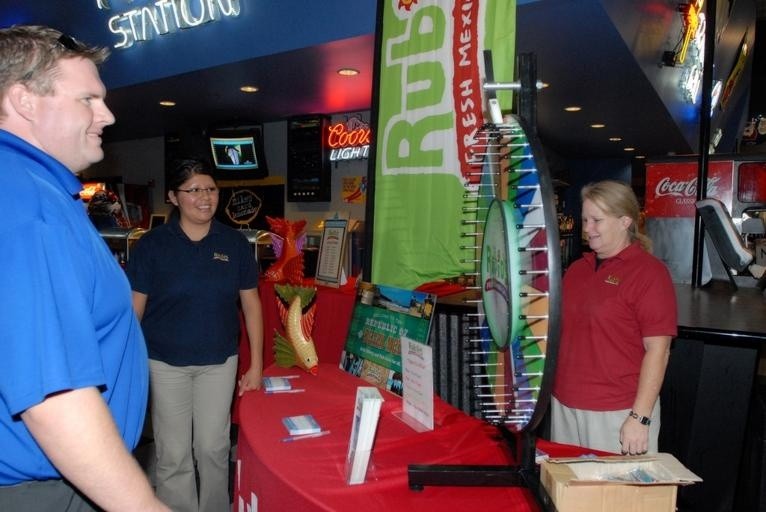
[280,429,331,442]
[260,375,306,394]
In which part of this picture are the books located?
[341,385,385,486]
[279,415,323,436]
[263,375,293,391]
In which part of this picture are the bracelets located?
[628,411,652,427]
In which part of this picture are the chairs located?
[696,198,766,289]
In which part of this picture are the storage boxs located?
[537,449,702,512]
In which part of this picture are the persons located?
[0,22,180,512]
[550,179,679,457]
[124,157,266,512]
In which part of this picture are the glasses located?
[178,188,218,196]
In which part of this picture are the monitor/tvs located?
[205,129,268,180]
[286,114,331,203]
